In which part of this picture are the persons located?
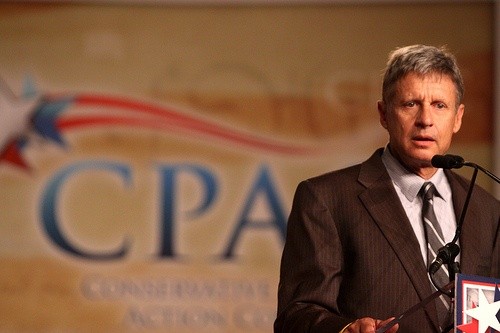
[273,44,500,333]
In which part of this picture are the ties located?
[418,181,457,332]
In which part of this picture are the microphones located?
[429,155,478,276]
[445,154,500,183]
